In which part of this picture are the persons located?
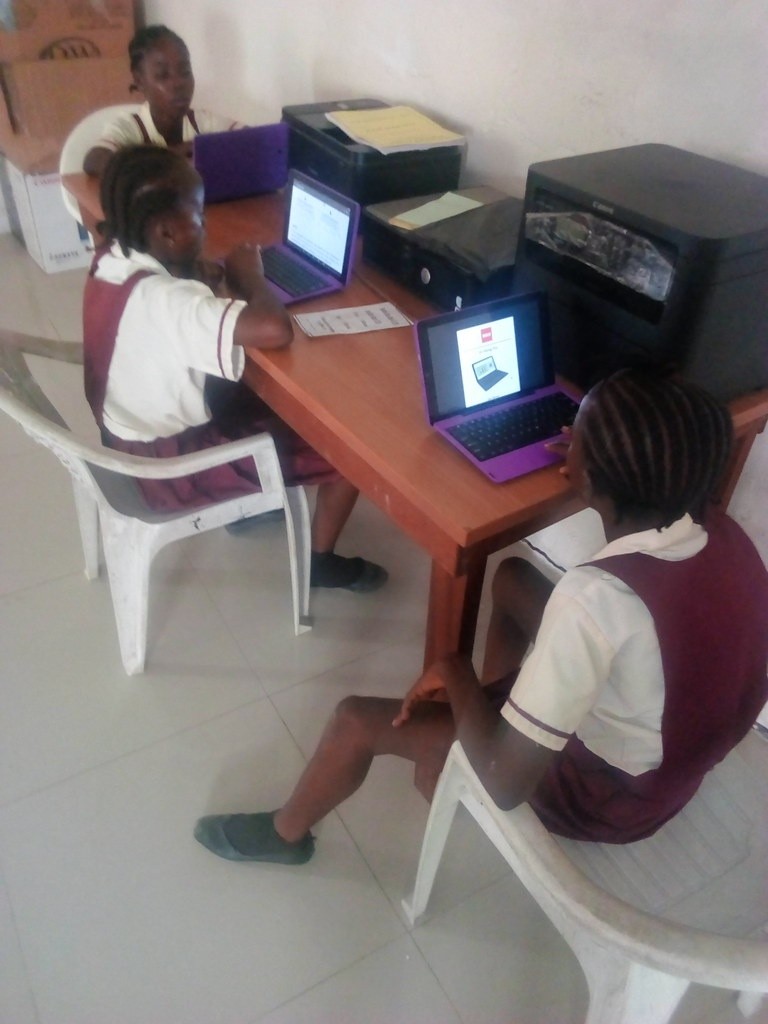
[81,23,256,175]
[80,146,390,592]
[192,370,767,866]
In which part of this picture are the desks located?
[61,156,768,803]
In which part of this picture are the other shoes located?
[310,553,389,592]
[193,814,315,865]
[224,480,296,531]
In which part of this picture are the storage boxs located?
[0,0,146,275]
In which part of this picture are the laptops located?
[191,120,291,206]
[211,167,360,302]
[413,287,585,486]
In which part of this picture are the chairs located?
[0,330,315,676]
[398,726,768,1024]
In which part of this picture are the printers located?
[366,184,526,311]
[516,142,767,410]
[278,96,464,209]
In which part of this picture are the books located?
[324,103,466,155]
